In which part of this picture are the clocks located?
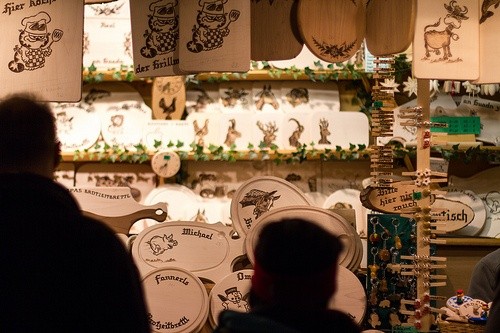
[151,152,181,177]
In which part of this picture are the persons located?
[210,217,365,332]
[0,92,152,333]
